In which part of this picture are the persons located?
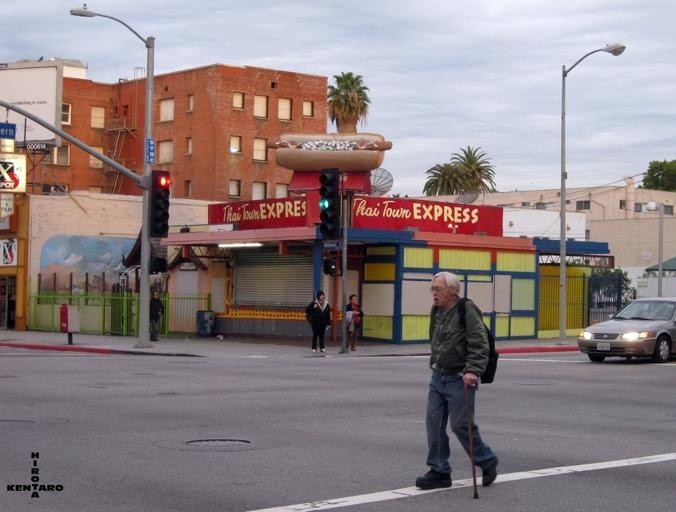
[7,293,16,329]
[309,291,330,355]
[347,294,363,351]
[414,271,500,490]
[151,290,165,341]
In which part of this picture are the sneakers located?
[480,456,498,486]
[416,470,452,489]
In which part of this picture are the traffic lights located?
[322,257,343,276]
[149,168,171,241]
[316,169,340,243]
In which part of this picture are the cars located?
[576,295,676,364]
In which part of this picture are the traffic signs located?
[144,137,156,166]
[0,122,17,139]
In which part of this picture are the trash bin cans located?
[196,310,215,337]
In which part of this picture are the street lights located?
[645,198,666,297]
[555,42,627,347]
[68,6,158,351]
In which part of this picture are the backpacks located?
[459,298,499,383]
